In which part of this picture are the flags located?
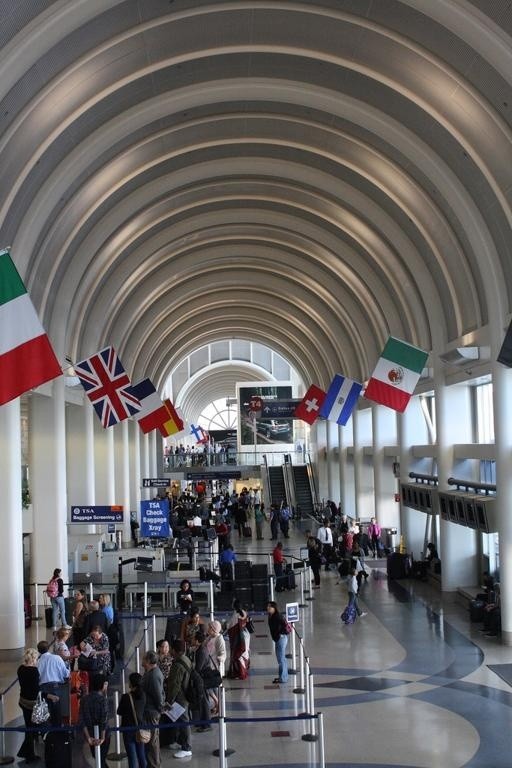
[318,372,363,426]
[72,344,144,429]
[158,397,209,444]
[133,377,171,434]
[292,383,327,426]
[363,335,430,413]
[0,250,64,406]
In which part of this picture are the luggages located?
[341,595,357,624]
[242,520,252,537]
[281,563,295,591]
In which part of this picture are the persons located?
[17,445,382,767]
[467,571,502,639]
[412,543,437,579]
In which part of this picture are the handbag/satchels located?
[135,726,152,744]
[187,666,223,711]
[47,579,59,598]
[246,618,255,633]
[31,692,51,725]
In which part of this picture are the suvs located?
[256,418,290,438]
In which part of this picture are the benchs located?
[427,569,441,582]
[456,585,484,610]
